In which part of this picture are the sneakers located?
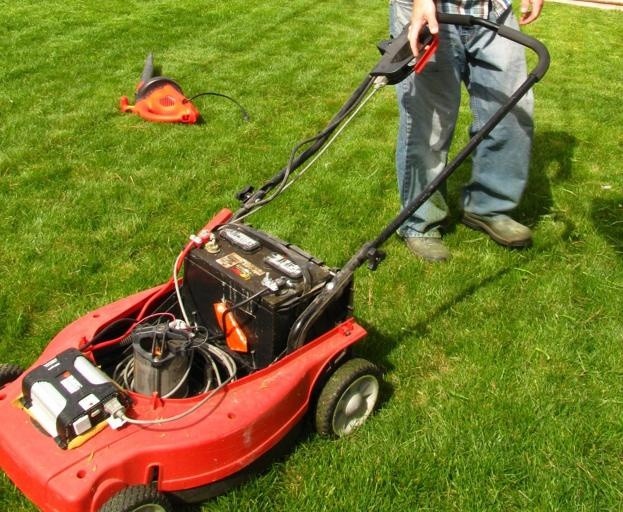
[405,233,454,264]
[461,209,532,246]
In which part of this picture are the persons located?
[387,0,547,263]
